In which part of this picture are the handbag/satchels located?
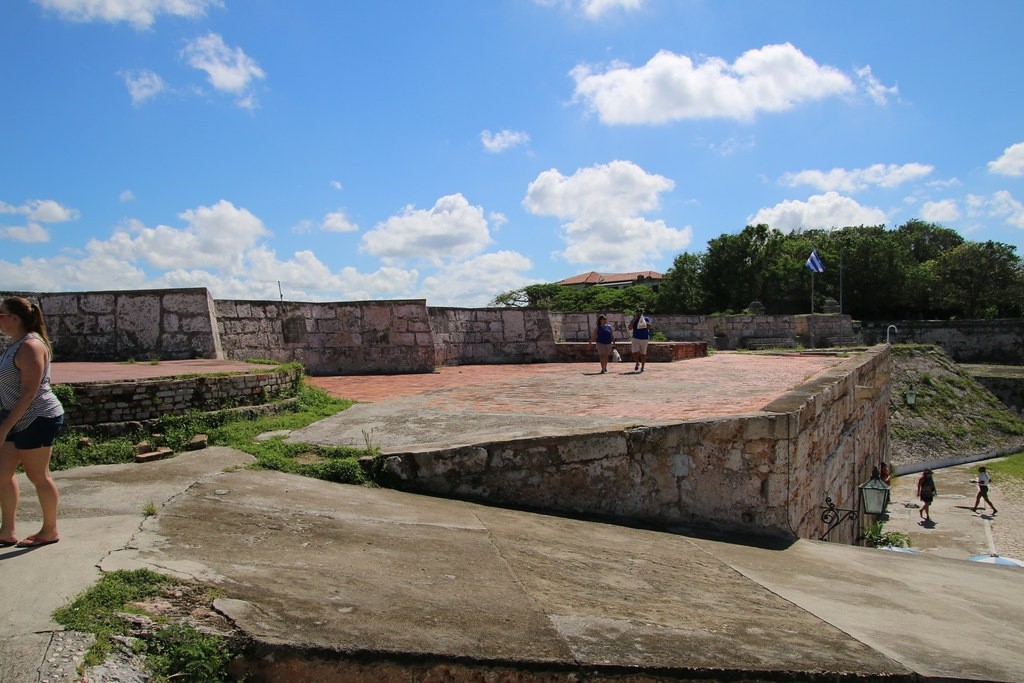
[637,315,647,329]
[612,348,622,362]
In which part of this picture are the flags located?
[806,249,825,274]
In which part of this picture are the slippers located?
[17,537,59,547]
[0,539,17,545]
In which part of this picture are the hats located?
[634,308,646,314]
[922,468,934,474]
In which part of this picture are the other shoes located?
[601,369,605,374]
[605,369,607,372]
[641,368,643,372]
[635,364,639,370]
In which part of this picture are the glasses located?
[603,318,607,320]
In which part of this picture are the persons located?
[881,461,891,513]
[918,468,937,519]
[0,296,66,547]
[628,309,652,371]
[969,467,998,514]
[588,315,616,373]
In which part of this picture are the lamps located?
[818,465,892,542]
